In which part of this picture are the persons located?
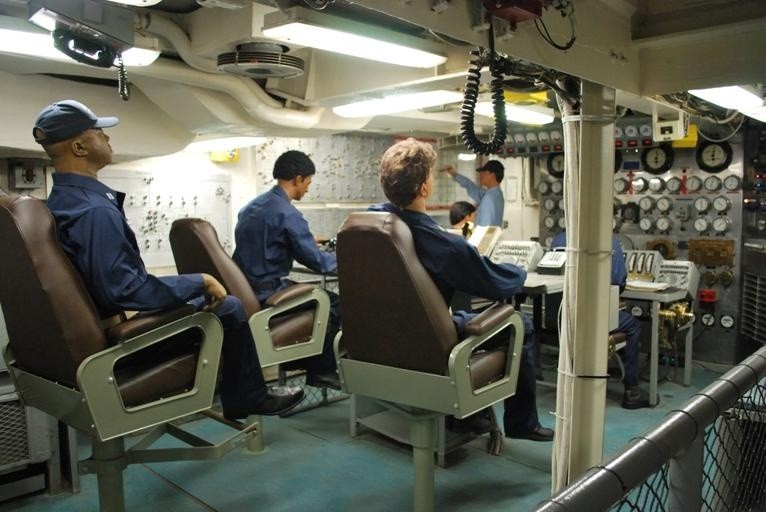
[443,160,504,225]
[448,201,477,228]
[31,98,308,422]
[368,136,554,442]
[230,150,342,391]
[544,203,660,410]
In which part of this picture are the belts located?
[102,311,139,330]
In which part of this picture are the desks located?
[620,284,688,409]
[517,271,567,382]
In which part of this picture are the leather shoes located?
[225,386,306,419]
[306,373,341,389]
[623,389,659,408]
[506,423,553,442]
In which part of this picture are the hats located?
[33,99,118,144]
[476,160,503,174]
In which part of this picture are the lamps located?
[263,5,447,70]
[470,98,556,128]
[687,84,766,112]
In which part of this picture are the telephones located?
[53,29,116,68]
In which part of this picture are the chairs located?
[324,207,525,512]
[166,215,340,454]
[0,192,259,511]
